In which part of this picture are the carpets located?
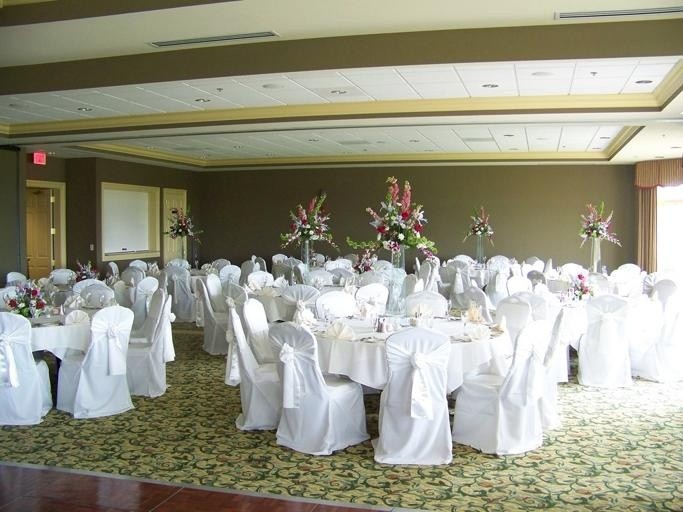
[0,327,683,512]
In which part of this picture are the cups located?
[322,304,329,315]
[325,313,335,325]
[561,291,568,301]
[569,289,574,298]
[100,294,107,304]
[461,311,469,331]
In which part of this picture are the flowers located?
[579,201,622,249]
[279,191,341,256]
[3,279,60,318]
[345,177,438,273]
[74,259,107,283]
[163,206,206,248]
[463,206,494,246]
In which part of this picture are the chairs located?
[51,270,78,293]
[446,261,471,308]
[80,285,114,308]
[358,273,384,286]
[514,291,550,330]
[579,296,631,389]
[73,280,106,298]
[644,272,663,296]
[402,274,418,297]
[316,292,360,320]
[282,284,320,321]
[494,295,531,369]
[405,292,447,317]
[195,278,229,356]
[560,263,583,279]
[383,267,407,288]
[324,261,343,271]
[335,259,352,271]
[220,265,240,289]
[330,268,354,286]
[269,322,362,454]
[229,306,283,431]
[206,274,228,313]
[356,284,388,318]
[248,272,274,290]
[540,306,566,426]
[6,273,27,286]
[295,263,307,285]
[284,258,303,282]
[163,267,197,323]
[305,270,333,287]
[244,299,278,365]
[222,283,247,339]
[126,295,171,395]
[108,262,121,285]
[616,263,642,296]
[255,258,267,273]
[542,257,553,275]
[314,254,324,266]
[273,261,293,287]
[239,260,256,286]
[485,255,510,305]
[532,261,544,273]
[380,329,451,464]
[415,257,419,277]
[534,283,560,308]
[120,267,146,287]
[650,279,679,308]
[453,320,553,457]
[129,261,147,274]
[167,258,189,269]
[373,261,393,271]
[454,255,474,268]
[273,255,287,265]
[0,312,51,423]
[418,262,432,292]
[129,288,165,343]
[134,277,158,326]
[212,259,230,274]
[345,254,357,269]
[0,287,24,309]
[526,256,538,265]
[589,271,609,296]
[452,286,493,322]
[62,307,134,417]
[507,275,529,296]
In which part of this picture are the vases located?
[476,236,486,264]
[391,245,405,270]
[300,240,313,267]
[177,238,187,260]
[589,237,604,277]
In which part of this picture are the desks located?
[3,309,96,362]
[560,296,665,372]
[299,316,503,397]
[441,267,496,290]
[257,286,345,322]
[153,270,219,298]
